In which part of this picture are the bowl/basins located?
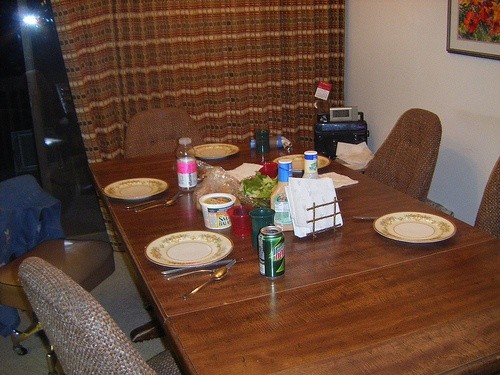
[199,193,236,229]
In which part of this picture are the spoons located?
[183,266,229,300]
[134,195,178,213]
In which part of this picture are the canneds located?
[258,226,285,278]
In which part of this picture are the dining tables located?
[89,142,500,375]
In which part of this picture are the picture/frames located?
[446,0,500,59]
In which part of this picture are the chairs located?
[0,175,117,375]
[126,109,205,156]
[360,108,441,201]
[18,257,185,375]
[473,155,500,238]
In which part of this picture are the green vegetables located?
[238,171,277,199]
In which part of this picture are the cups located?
[255,130,270,152]
[255,162,278,178]
[249,208,275,251]
[227,206,255,235]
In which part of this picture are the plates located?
[272,154,329,172]
[188,143,240,160]
[373,212,457,243]
[104,178,168,201]
[144,230,233,268]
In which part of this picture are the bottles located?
[303,151,321,179]
[175,138,197,191]
[270,160,292,232]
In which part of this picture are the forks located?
[165,258,236,280]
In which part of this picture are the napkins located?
[283,171,359,238]
[229,161,262,181]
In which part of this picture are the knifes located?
[161,258,246,275]
[124,194,183,209]
